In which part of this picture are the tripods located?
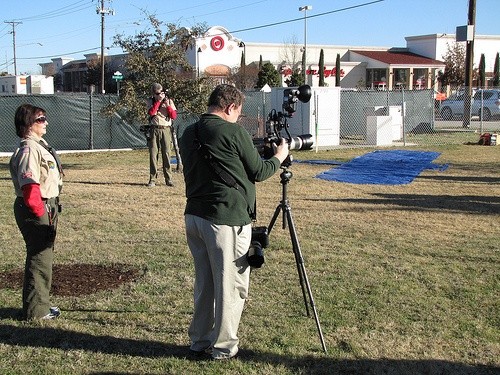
[267,153,328,354]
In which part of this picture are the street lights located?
[299,6,313,86]
[13,43,44,75]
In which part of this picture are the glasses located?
[235,109,243,122]
[154,92,160,96]
[33,116,48,124]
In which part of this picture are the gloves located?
[36,211,50,230]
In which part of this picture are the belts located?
[151,125,171,129]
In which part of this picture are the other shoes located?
[39,306,61,320]
[233,347,254,360]
[166,182,175,187]
[148,180,155,187]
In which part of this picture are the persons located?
[176,82,290,360]
[8,104,65,320]
[148,81,176,189]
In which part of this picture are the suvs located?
[439,89,500,121]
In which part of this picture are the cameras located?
[163,88,173,98]
[263,134,313,159]
[247,226,269,268]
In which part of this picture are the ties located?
[39,139,64,175]
